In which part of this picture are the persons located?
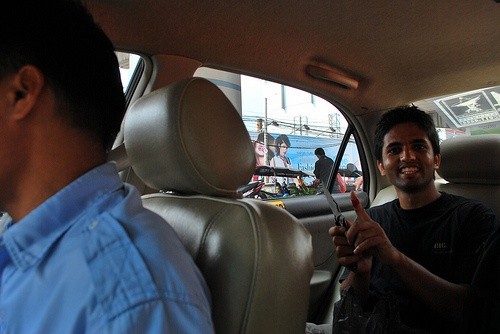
[329,105,500,334]
[270,134,298,188]
[313,148,340,194]
[251,133,276,184]
[0,0,215,334]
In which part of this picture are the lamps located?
[257,121,279,133]
[290,124,310,135]
[316,127,336,137]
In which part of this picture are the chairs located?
[124,77,314,334]
[327,135,500,334]
